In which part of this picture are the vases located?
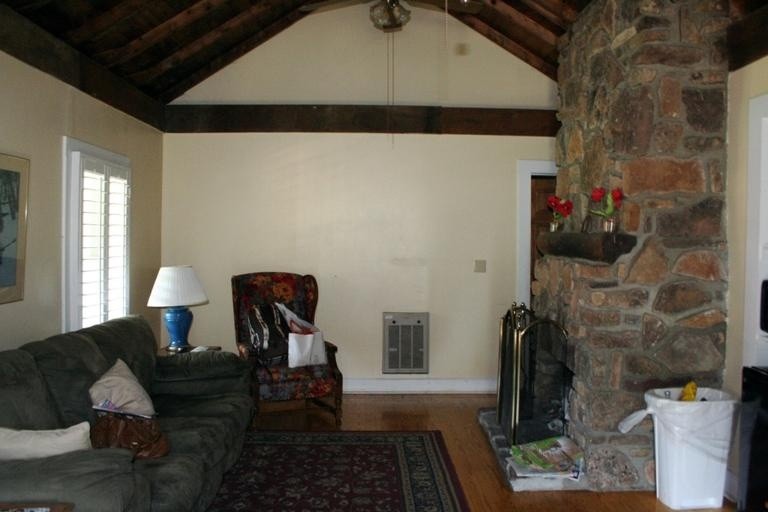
[549,219,560,232]
[604,218,614,233]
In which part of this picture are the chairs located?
[230,272,342,425]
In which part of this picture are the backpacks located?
[246,304,291,365]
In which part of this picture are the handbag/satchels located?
[289,330,328,368]
[93,412,171,458]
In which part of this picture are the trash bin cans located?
[645,385,736,509]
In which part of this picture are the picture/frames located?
[0,151,31,304]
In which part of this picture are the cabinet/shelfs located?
[156,346,222,357]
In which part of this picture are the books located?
[507,434,584,477]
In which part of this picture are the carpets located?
[210,428,470,512]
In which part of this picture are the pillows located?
[88,359,153,417]
[0,421,92,461]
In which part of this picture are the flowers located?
[588,187,622,217]
[547,196,574,216]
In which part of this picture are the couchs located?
[0,316,255,512]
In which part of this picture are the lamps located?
[370,0,411,33]
[148,263,210,351]
